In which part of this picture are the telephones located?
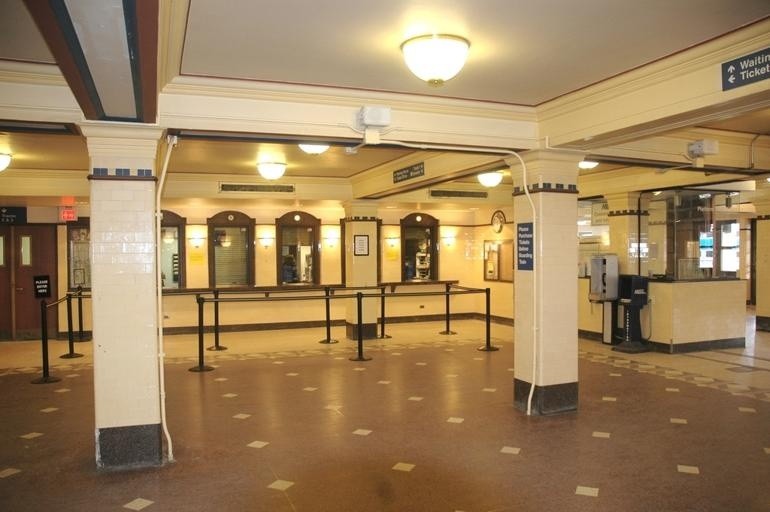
[602,273,606,286]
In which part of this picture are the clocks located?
[493,215,504,233]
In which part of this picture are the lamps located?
[1,154,10,172]
[220,242,231,247]
[579,161,600,169]
[476,170,504,187]
[400,34,470,88]
[298,142,330,155]
[256,163,286,180]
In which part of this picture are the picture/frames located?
[354,235,369,256]
